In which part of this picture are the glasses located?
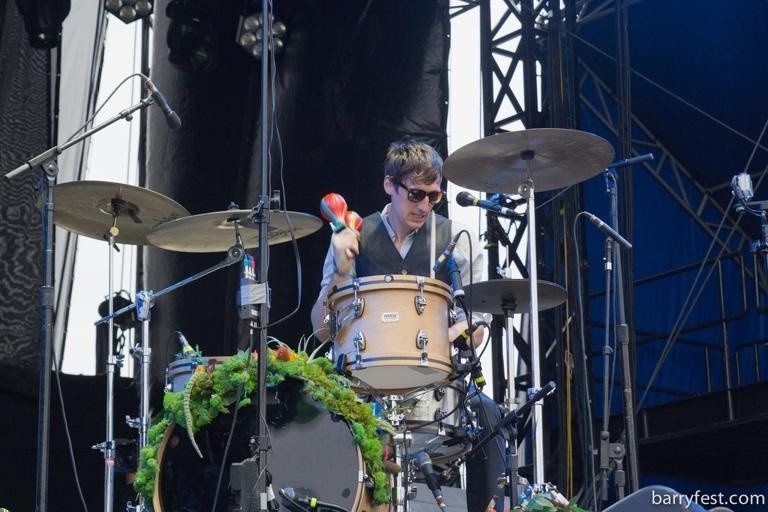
[399,182,445,204]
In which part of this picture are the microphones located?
[590,214,631,249]
[145,80,181,129]
[454,322,480,349]
[417,452,446,510]
[456,191,518,219]
[433,232,460,271]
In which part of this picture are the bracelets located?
[332,257,346,278]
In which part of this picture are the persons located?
[308,138,507,511]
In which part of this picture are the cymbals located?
[147,209,323,255]
[456,280,568,315]
[35,181,189,245]
[442,128,615,193]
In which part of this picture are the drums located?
[376,379,474,464]
[153,375,394,512]
[327,275,454,394]
